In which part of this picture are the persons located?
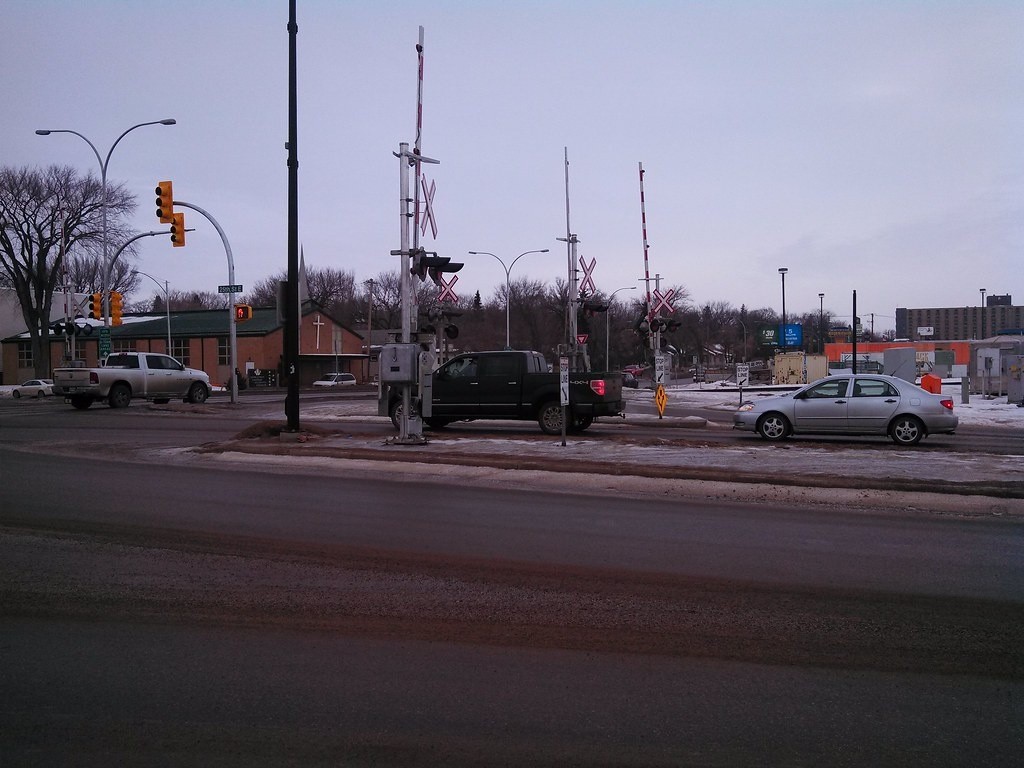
[814,382,848,397]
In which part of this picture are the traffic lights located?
[171,212,187,247]
[155,181,173,224]
[235,304,253,321]
[88,293,101,319]
[428,261,465,286]
[650,319,663,332]
[413,249,451,281]
[668,320,682,332]
[444,324,459,338]
[422,324,436,333]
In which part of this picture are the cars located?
[10,379,56,399]
[733,373,959,447]
[617,362,655,388]
[692,372,706,381]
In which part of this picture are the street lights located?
[468,249,549,348]
[131,269,173,368]
[587,286,637,371]
[817,293,825,349]
[979,288,987,342]
[32,117,177,359]
[777,268,790,353]
[720,314,747,364]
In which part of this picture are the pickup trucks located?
[50,351,213,408]
[389,351,626,436]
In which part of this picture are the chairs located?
[854,384,861,397]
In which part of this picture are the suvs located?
[313,373,356,387]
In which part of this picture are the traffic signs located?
[100,342,110,356]
[218,284,242,293]
[98,327,112,343]
[736,364,750,386]
